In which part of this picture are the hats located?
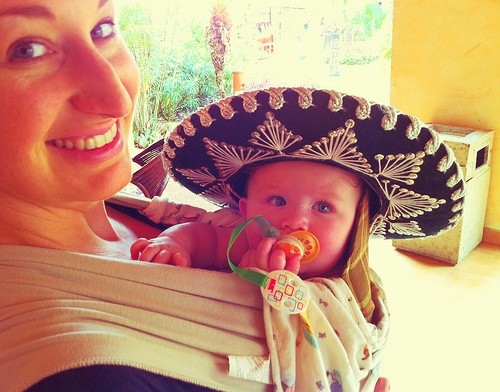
[162,86,467,240]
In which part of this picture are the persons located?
[0,0,393,392]
[130,85,465,323]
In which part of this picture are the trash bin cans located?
[385,123,494,264]
